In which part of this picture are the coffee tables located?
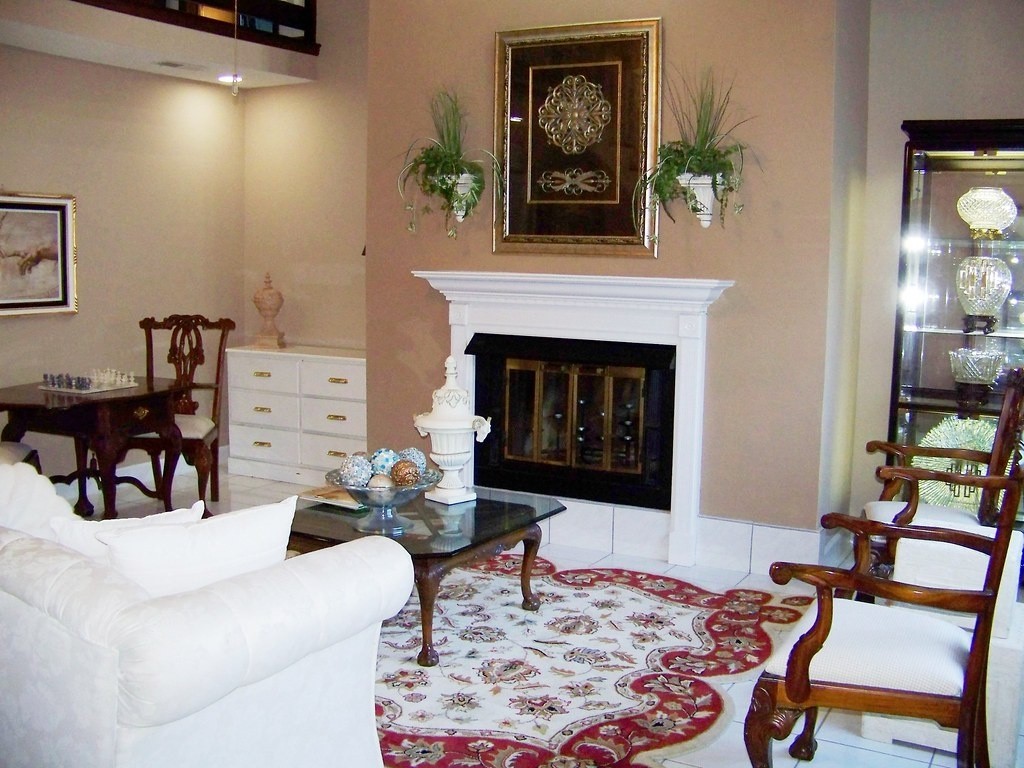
[290,485,568,667]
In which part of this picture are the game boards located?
[36,379,139,395]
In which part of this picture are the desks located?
[0,377,183,518]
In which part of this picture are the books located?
[298,486,368,510]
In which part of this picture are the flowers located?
[629,53,765,247]
[396,87,494,239]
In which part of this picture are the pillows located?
[99,492,299,597]
[48,500,204,558]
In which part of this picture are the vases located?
[426,173,471,222]
[674,171,724,229]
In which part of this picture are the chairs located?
[138,313,236,510]
[734,365,1024,768]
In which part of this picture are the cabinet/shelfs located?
[225,344,366,486]
[885,114,1024,529]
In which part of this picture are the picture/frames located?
[0,193,79,318]
[492,15,662,261]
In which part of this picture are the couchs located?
[0,462,415,768]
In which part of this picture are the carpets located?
[375,546,816,768]
